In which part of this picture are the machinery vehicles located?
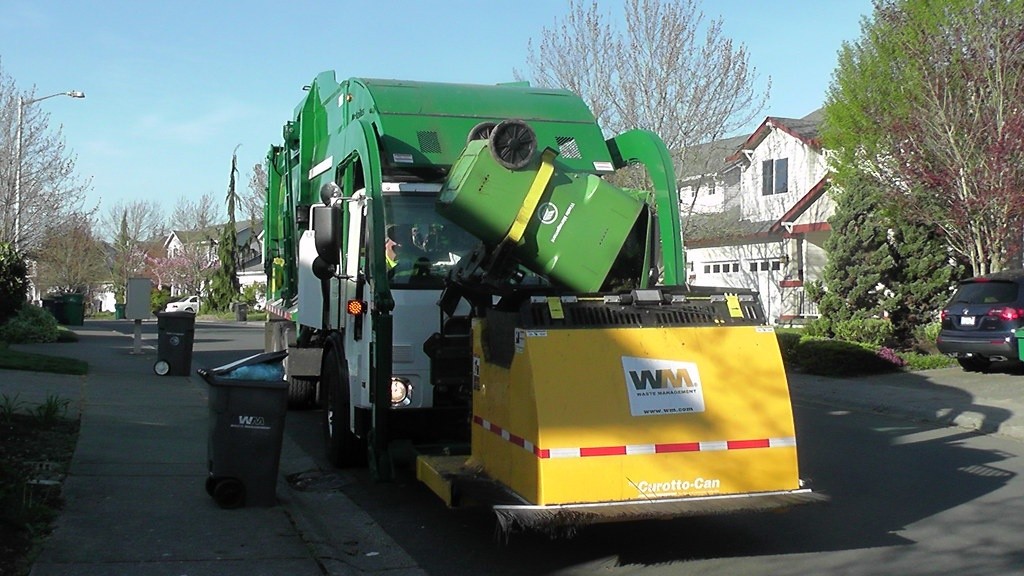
[261,67,829,545]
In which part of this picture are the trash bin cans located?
[434,118,646,297]
[62,293,85,327]
[235,302,247,323]
[152,310,196,378]
[115,303,127,320]
[197,348,293,511]
[40,297,65,325]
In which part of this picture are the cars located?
[164,295,204,312]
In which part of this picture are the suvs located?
[935,267,1024,373]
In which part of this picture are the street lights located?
[14,89,87,252]
